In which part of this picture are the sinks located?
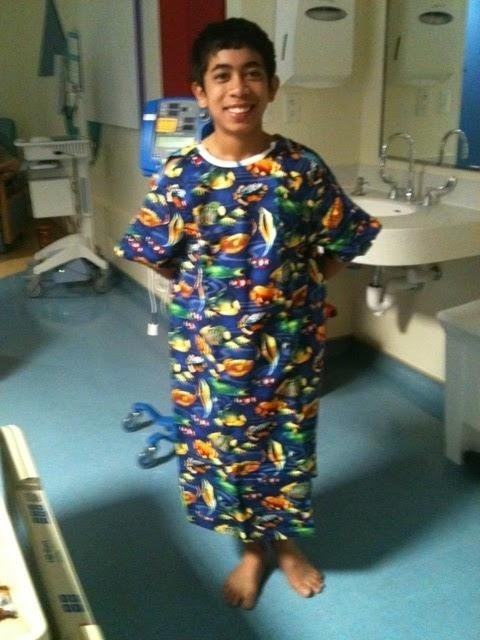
[350,198,416,218]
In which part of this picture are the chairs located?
[0,118,25,248]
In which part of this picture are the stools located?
[434,300,480,466]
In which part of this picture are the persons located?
[111,18,382,610]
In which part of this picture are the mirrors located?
[379,0,480,170]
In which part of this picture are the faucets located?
[377,132,416,202]
[437,129,469,165]
